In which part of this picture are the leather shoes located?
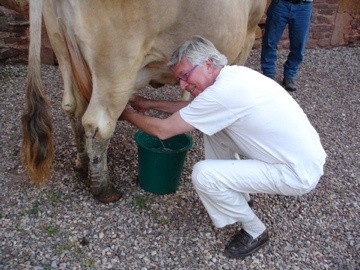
[224,227,271,256]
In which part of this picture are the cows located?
[20,0,267,203]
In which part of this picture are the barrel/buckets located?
[134,128,193,194]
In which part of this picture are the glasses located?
[175,60,198,85]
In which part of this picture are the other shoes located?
[282,80,297,91]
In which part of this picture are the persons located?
[260,0,314,91]
[121,35,329,258]
